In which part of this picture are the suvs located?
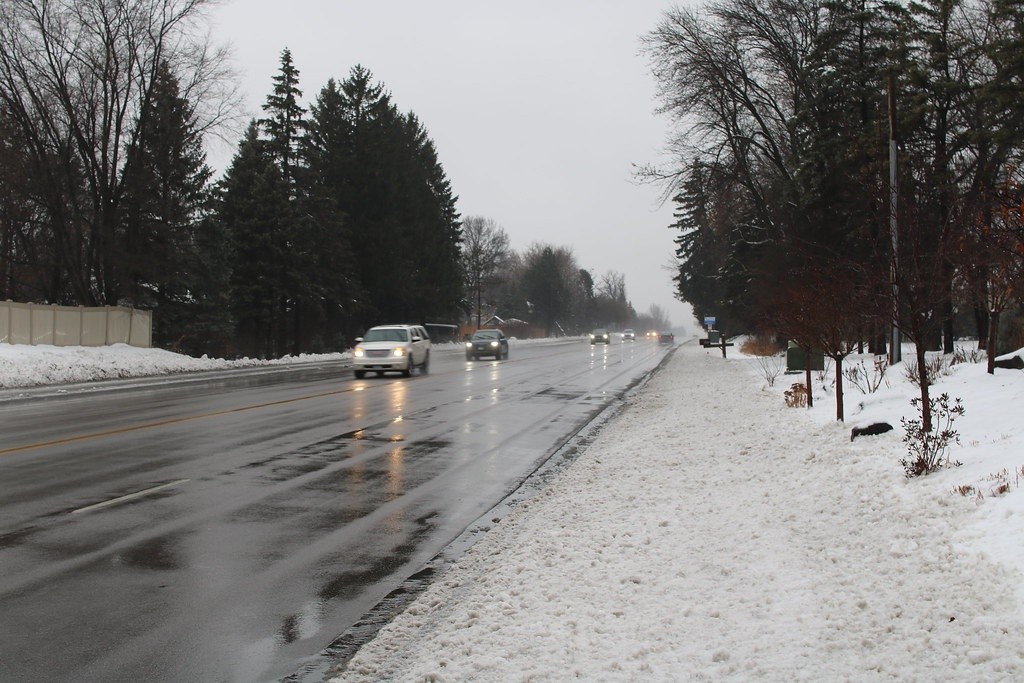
[352,324,431,379]
[465,329,509,360]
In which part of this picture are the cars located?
[658,333,673,343]
[591,329,610,344]
[622,329,635,340]
